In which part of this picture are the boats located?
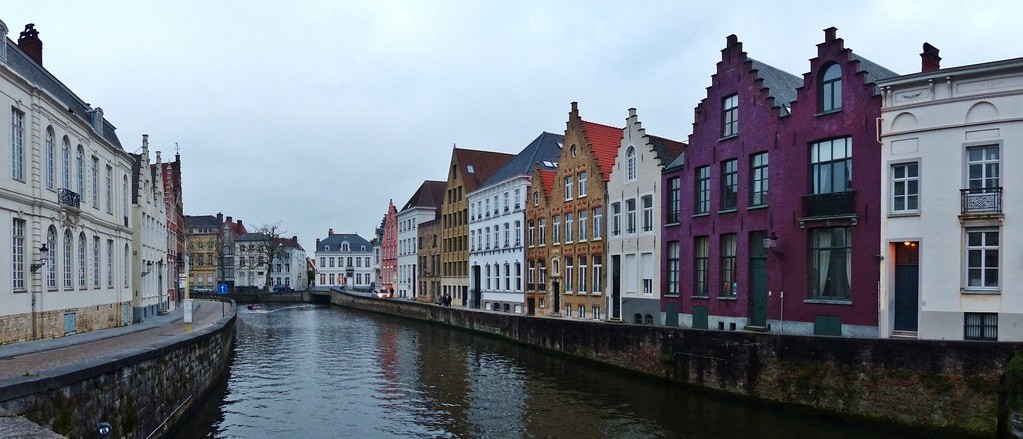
[248,304,264,311]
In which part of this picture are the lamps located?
[761,231,781,258]
[147,260,152,273]
[31,244,49,272]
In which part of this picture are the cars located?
[371,289,390,298]
[273,284,295,291]
[194,286,212,293]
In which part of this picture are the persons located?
[390,288,394,294]
[438,293,452,307]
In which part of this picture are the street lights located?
[271,243,282,292]
